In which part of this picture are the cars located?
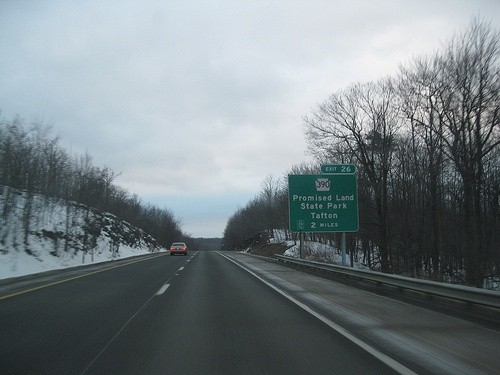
[170,242,187,256]
